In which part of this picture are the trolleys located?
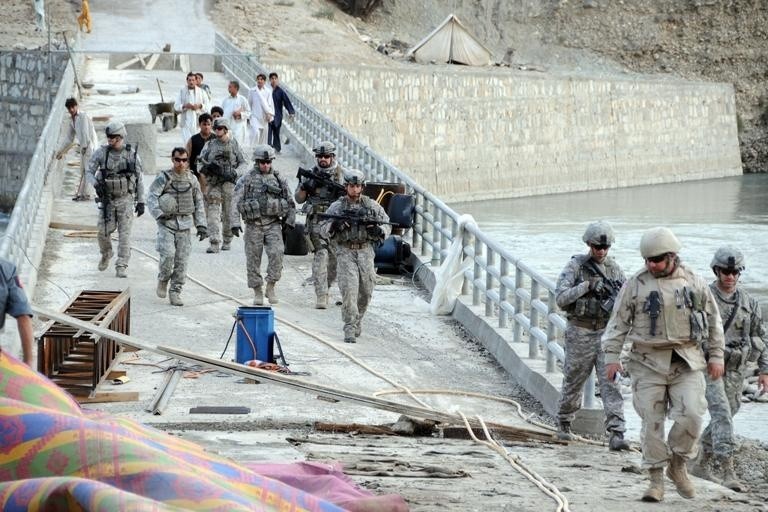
[147,101,184,133]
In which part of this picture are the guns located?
[580,256,623,312]
[95,178,110,237]
[317,208,399,236]
[197,154,236,185]
[296,167,347,201]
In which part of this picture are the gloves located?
[589,280,603,292]
[368,226,381,237]
[196,227,208,241]
[334,217,351,233]
[134,203,144,216]
[232,227,242,236]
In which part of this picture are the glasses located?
[648,252,672,262]
[720,268,739,275]
[591,246,608,250]
[173,158,187,162]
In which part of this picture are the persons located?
[57,98,97,201]
[554,222,630,451]
[84,122,145,278]
[0,258,34,368]
[324,169,392,342]
[32,0,45,34]
[146,147,208,306]
[185,107,250,252]
[78,0,91,33]
[601,228,725,503]
[293,140,350,308]
[174,73,296,154]
[232,145,297,305]
[690,244,768,491]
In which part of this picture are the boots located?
[722,458,741,492]
[666,453,696,499]
[253,286,263,304]
[265,282,277,304]
[643,469,663,501]
[689,446,712,480]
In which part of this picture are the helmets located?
[159,194,178,213]
[640,228,682,259]
[710,245,745,272]
[255,145,275,160]
[582,222,615,246]
[105,121,127,138]
[344,169,365,184]
[315,141,335,155]
[214,118,229,130]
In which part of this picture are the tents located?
[407,13,495,67]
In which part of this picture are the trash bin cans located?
[234,304,275,367]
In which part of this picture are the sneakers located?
[169,291,183,306]
[157,281,167,297]
[98,252,114,270]
[117,266,127,277]
[610,435,629,451]
[344,327,361,342]
[222,241,231,250]
[316,295,327,308]
[558,422,571,440]
[206,242,219,252]
